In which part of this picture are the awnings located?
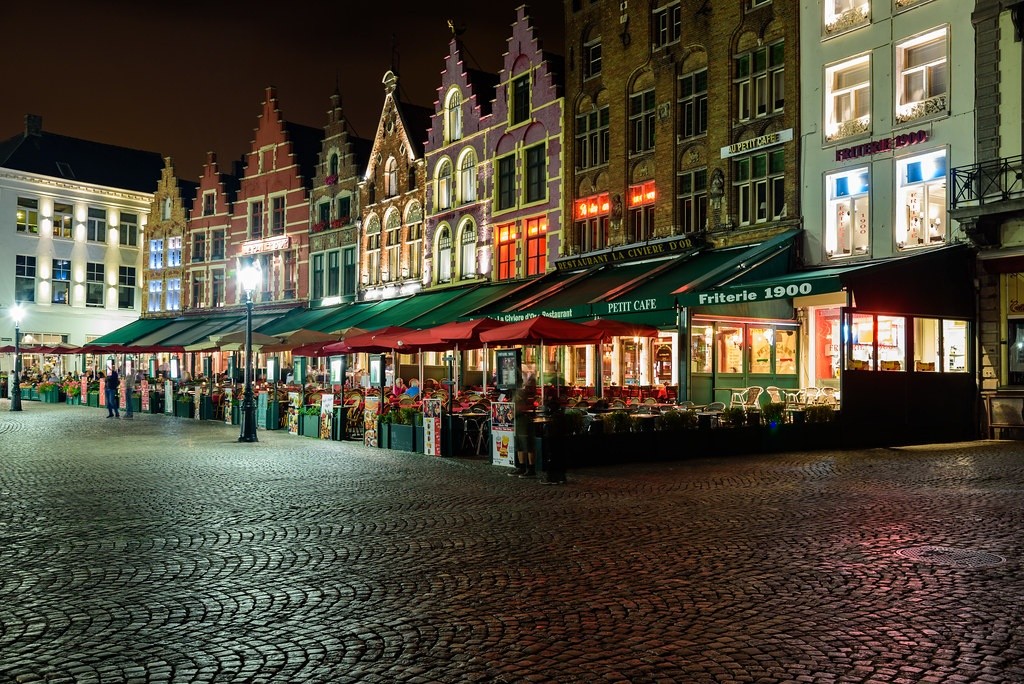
[676,242,976,312]
[84,229,802,349]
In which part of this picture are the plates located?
[366,411,377,447]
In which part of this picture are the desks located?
[525,409,545,417]
[630,402,676,412]
[712,387,749,409]
[765,387,803,422]
[605,407,631,424]
[458,412,488,456]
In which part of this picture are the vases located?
[65,394,80,405]
[39,387,58,404]
[20,388,31,400]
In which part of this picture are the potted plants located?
[30,388,41,401]
[129,393,141,413]
[377,407,424,454]
[298,407,321,438]
[174,394,194,418]
[90,391,100,408]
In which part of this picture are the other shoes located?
[115,415,121,418]
[123,415,133,420]
[107,414,114,418]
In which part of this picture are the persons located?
[395,376,419,391]
[104,371,121,418]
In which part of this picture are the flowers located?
[19,383,32,388]
[63,381,80,397]
[311,222,326,233]
[37,381,62,393]
[322,175,338,185]
[332,216,348,228]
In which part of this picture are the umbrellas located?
[0,315,659,412]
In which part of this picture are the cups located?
[495,436,501,450]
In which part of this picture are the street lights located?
[237,256,263,443]
[9,306,26,412]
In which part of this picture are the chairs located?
[729,385,840,422]
[209,384,726,455]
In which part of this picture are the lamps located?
[935,218,941,230]
[919,211,924,223]
